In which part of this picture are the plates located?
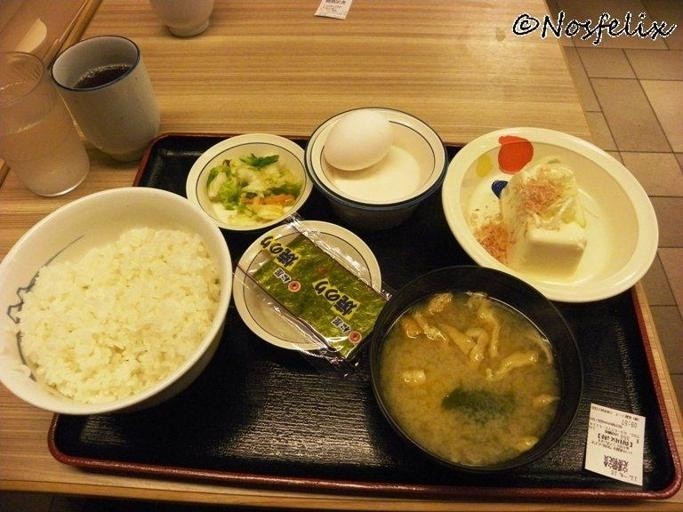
[233,219,384,355]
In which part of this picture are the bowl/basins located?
[185,131,315,232]
[306,106,448,212]
[368,265,586,473]
[441,124,661,305]
[0,184,234,417]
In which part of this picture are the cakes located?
[500,163,588,280]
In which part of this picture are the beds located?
[0,0,683,512]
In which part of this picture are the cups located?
[0,51,90,198]
[50,34,161,164]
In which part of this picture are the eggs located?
[325,109,392,172]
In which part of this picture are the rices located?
[0,226,222,407]
